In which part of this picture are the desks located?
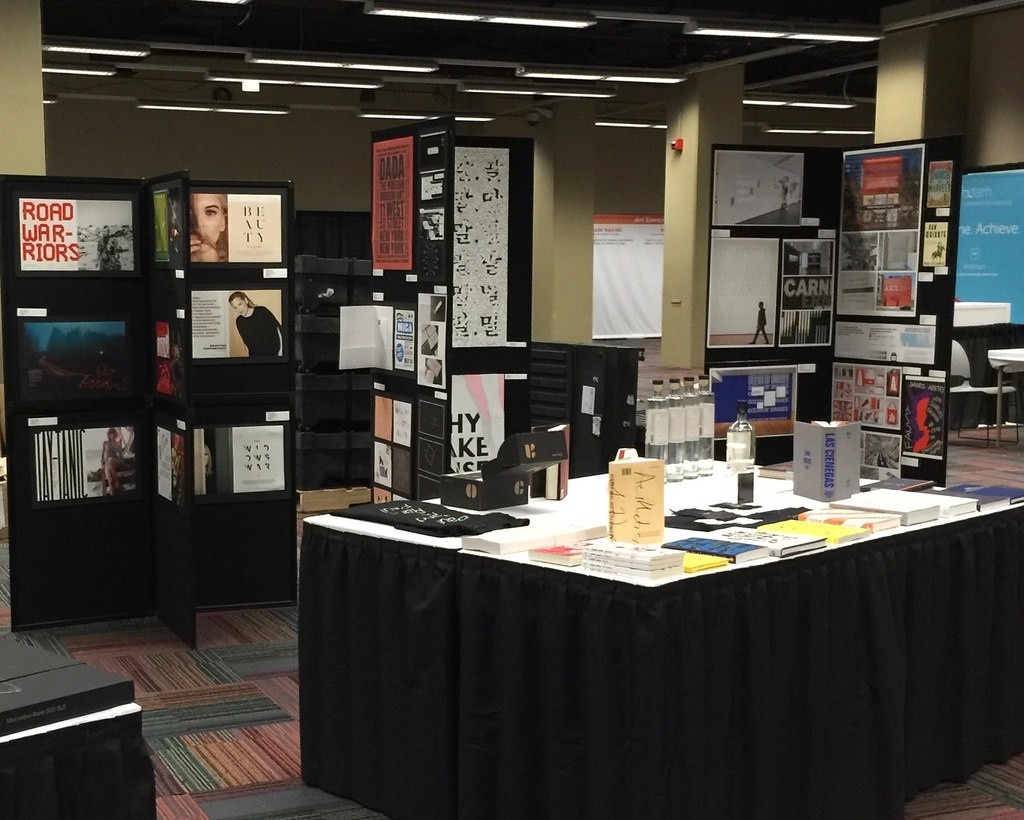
[0,702,156,820]
[988,348,1024,448]
[299,460,1024,820]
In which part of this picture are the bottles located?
[725,400,756,469]
[644,374,715,482]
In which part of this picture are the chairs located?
[950,337,1019,448]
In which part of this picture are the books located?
[462,477,1024,581]
[0,633,137,739]
[157,414,288,505]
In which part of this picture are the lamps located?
[458,81,617,98]
[42,61,138,78]
[242,50,441,74]
[595,117,669,130]
[762,124,875,136]
[743,95,857,108]
[43,93,58,106]
[137,99,292,115]
[364,0,597,29]
[515,63,689,84]
[41,38,153,58]
[682,17,884,44]
[203,69,386,89]
[361,108,495,124]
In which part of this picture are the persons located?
[779,180,789,210]
[189,193,229,263]
[229,291,283,357]
[172,435,183,501]
[204,444,214,474]
[97,225,121,270]
[749,300,770,344]
[101,427,128,496]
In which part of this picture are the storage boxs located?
[294,253,374,490]
[440,430,568,510]
